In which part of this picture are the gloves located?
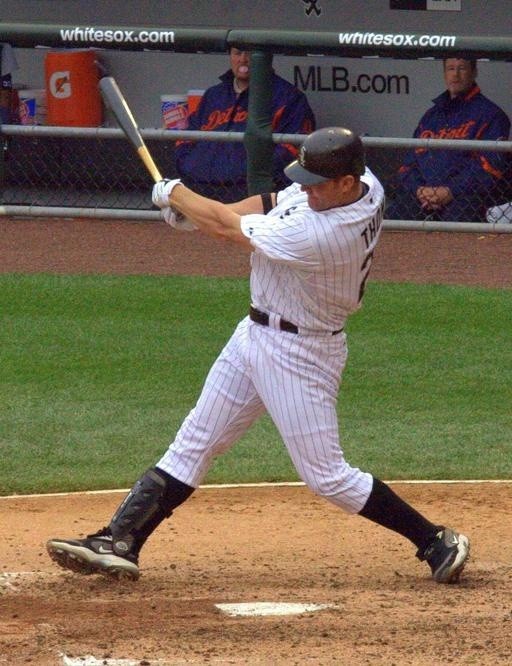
[160,206,200,232]
[151,178,181,208]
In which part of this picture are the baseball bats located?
[97,77,185,222]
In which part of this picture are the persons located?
[0,42,16,203]
[385,54,512,223]
[44,125,471,584]
[148,46,318,209]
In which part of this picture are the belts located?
[249,302,344,336]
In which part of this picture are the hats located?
[283,126,366,186]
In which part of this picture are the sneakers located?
[415,525,470,583]
[46,527,140,582]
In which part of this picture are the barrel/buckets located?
[17,90,48,125]
[160,94,188,130]
[44,47,106,126]
[187,87,205,116]
